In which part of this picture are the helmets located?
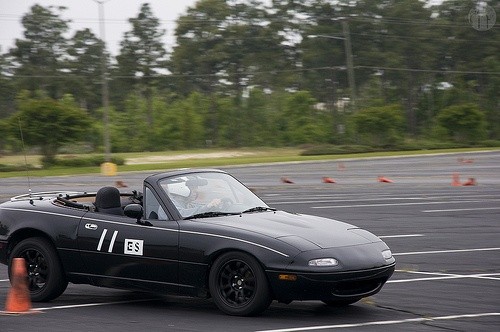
[167,176,208,200]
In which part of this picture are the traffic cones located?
[278,151,479,188]
[1,257,46,316]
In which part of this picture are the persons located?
[162,177,222,219]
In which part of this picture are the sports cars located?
[0,166,396,316]
